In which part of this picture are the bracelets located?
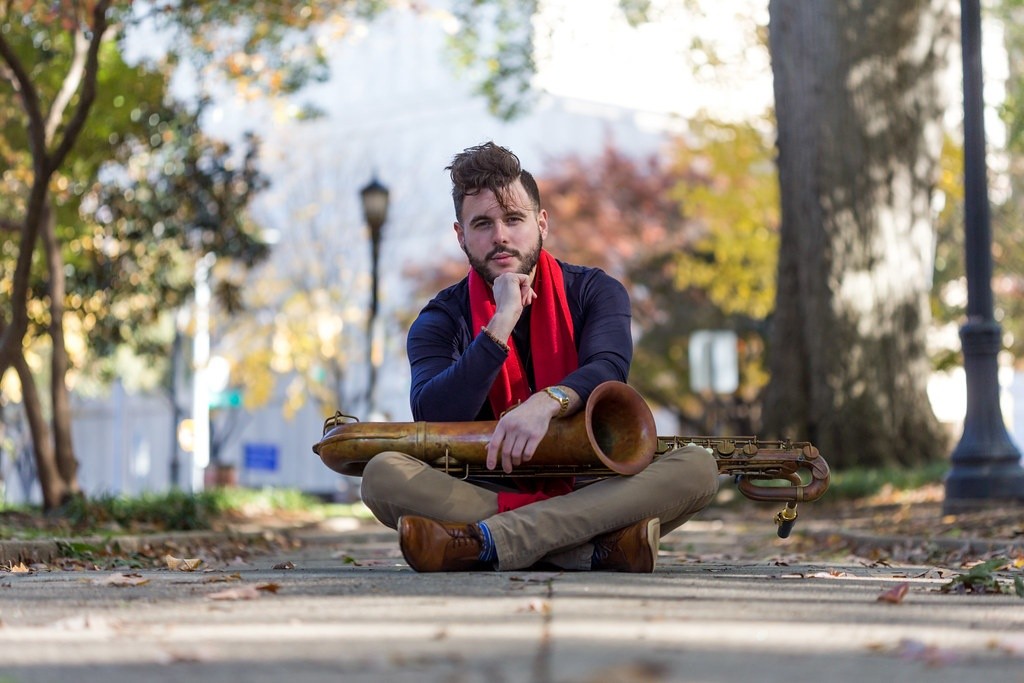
[481,326,510,351]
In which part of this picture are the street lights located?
[358,171,390,411]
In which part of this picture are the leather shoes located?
[398,515,485,573]
[591,517,660,574]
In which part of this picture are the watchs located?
[540,386,570,420]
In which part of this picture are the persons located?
[17,446,35,504]
[362,140,720,575]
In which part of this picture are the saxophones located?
[311,380,833,540]
